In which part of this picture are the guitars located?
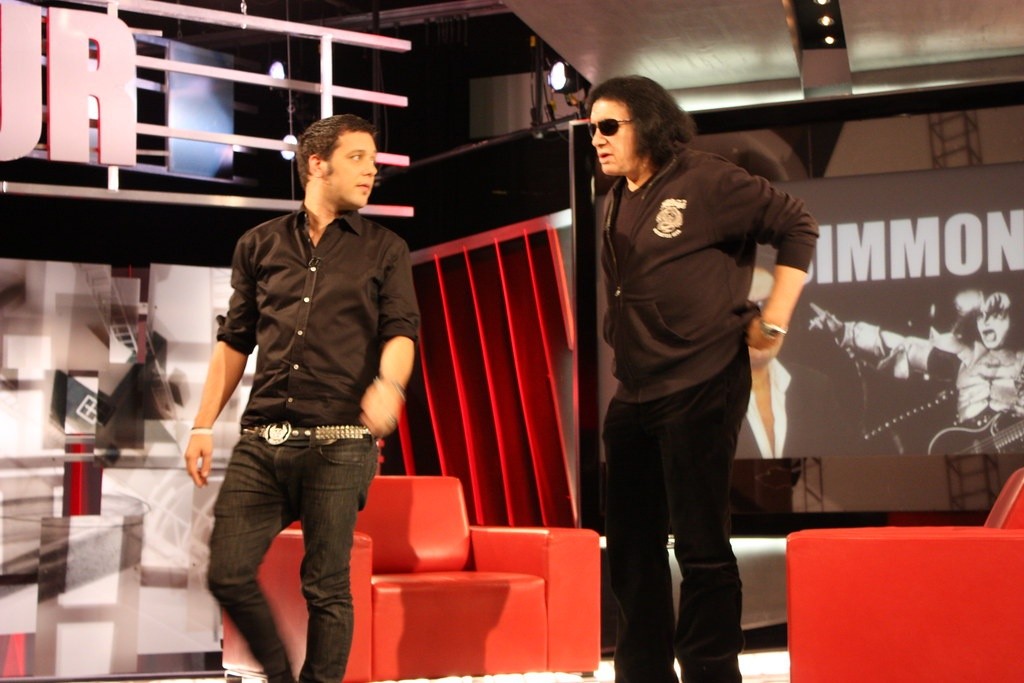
[928,410,1024,454]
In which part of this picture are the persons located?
[732,253,838,458]
[588,75,819,683]
[804,286,1024,454]
[184,114,420,683]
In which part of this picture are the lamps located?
[547,60,591,95]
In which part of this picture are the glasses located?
[588,118,635,138]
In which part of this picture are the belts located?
[242,419,372,445]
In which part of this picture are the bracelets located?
[190,427,212,434]
[373,378,407,397]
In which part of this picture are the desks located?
[602,537,792,651]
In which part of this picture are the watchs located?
[758,320,785,340]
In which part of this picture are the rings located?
[388,415,396,425]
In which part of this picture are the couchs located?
[223,476,601,683]
[788,468,1024,683]
